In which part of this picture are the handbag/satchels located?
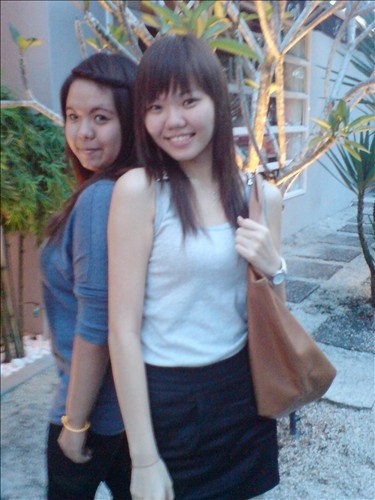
[245,171,338,418]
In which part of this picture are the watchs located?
[271,257,288,288]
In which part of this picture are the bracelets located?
[59,416,91,433]
[131,458,163,468]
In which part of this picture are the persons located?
[38,52,138,500]
[107,35,286,500]
